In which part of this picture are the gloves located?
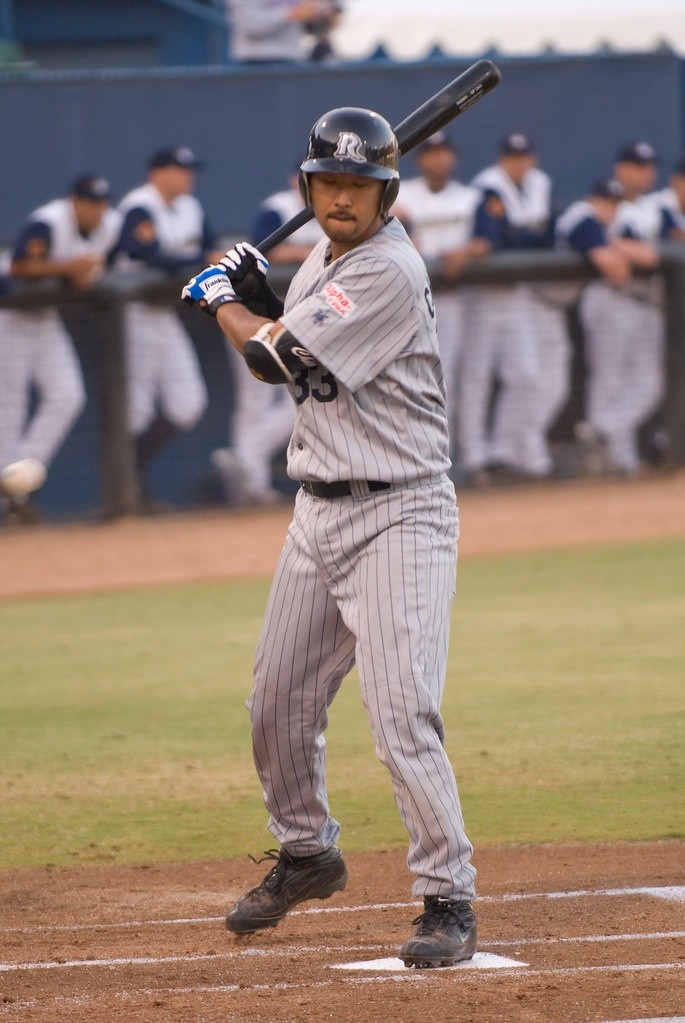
[180,262,238,318]
[217,242,269,303]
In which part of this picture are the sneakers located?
[225,849,349,932]
[399,896,477,968]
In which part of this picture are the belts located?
[303,481,391,498]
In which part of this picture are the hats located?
[421,133,449,148]
[616,140,665,168]
[72,175,111,197]
[504,136,532,151]
[149,147,203,169]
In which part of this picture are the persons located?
[181,105,477,971]
[576,135,685,479]
[112,144,211,469]
[390,123,498,470]
[456,133,562,475]
[0,169,131,526]
[522,178,632,429]
[204,151,327,501]
[230,0,340,63]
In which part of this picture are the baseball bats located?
[184,54,503,309]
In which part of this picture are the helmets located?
[298,107,400,219]
